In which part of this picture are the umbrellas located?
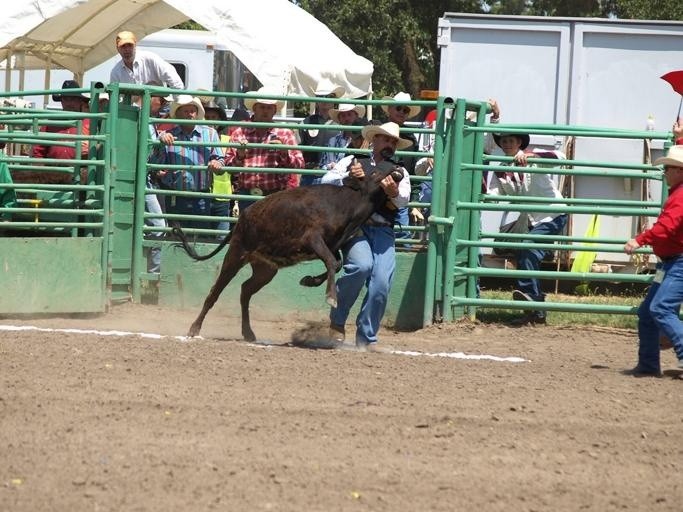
[659,70,683,122]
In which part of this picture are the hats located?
[329,104,366,123]
[53,81,90,101]
[116,31,136,47]
[655,145,683,167]
[362,122,413,149]
[227,108,253,121]
[145,81,227,131]
[492,131,529,150]
[310,78,345,98]
[243,88,285,113]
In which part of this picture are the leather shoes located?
[330,321,368,351]
[514,291,545,324]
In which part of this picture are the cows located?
[166,156,405,343]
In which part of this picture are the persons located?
[623,116,682,377]
[319,121,413,349]
[414,98,500,298]
[130,80,306,275]
[298,79,436,246]
[493,131,570,326]
[109,30,185,114]
[0,79,99,221]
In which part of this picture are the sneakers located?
[622,361,683,377]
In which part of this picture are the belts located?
[365,220,395,228]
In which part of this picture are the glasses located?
[316,95,334,98]
[665,167,680,172]
[396,106,409,113]
[381,92,421,119]
